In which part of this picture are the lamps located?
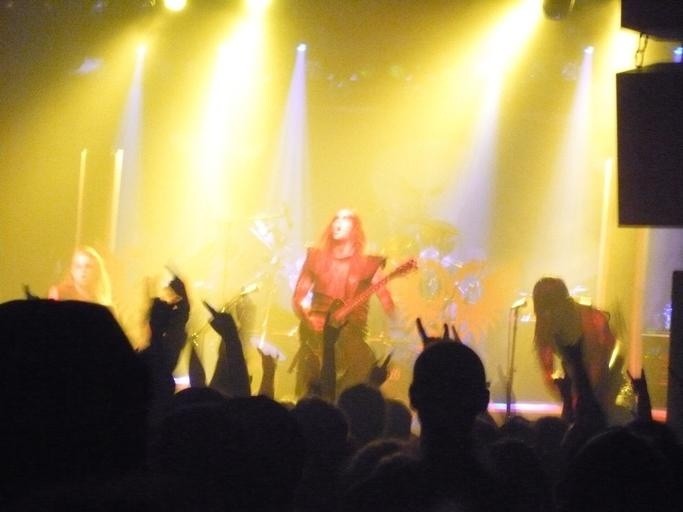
[543,0,575,21]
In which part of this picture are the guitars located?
[300,261,417,337]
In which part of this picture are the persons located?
[44,247,113,302]
[1,263,682,511]
[291,209,396,403]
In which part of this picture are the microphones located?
[241,284,258,297]
[512,300,527,309]
